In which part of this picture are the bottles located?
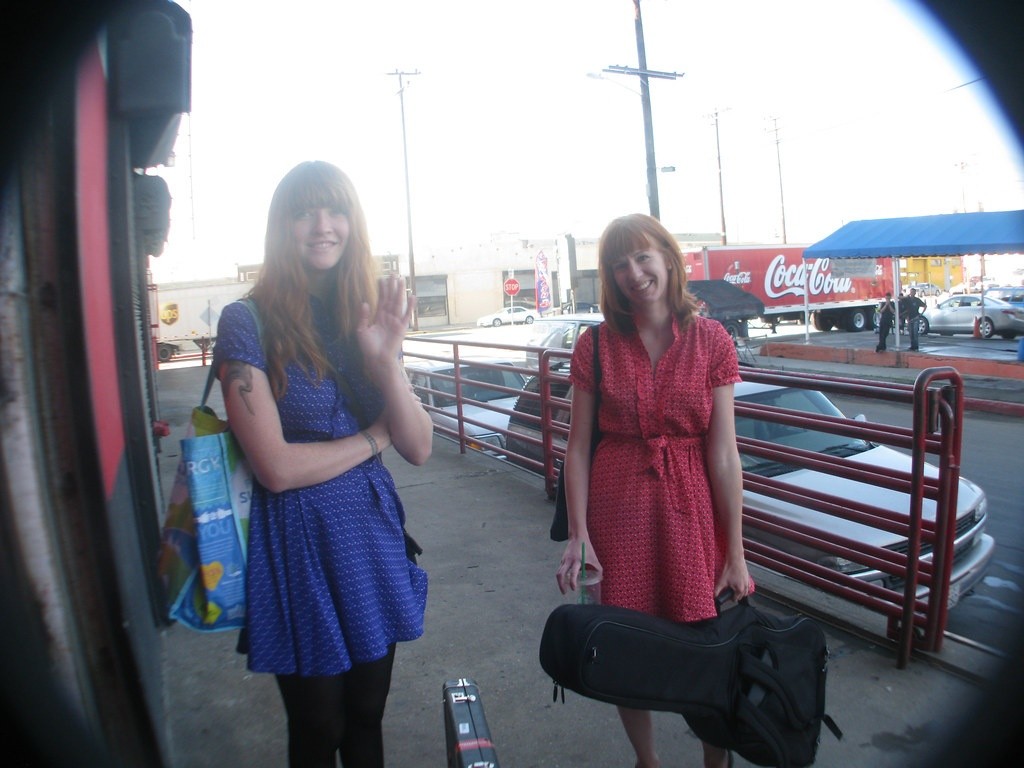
[734,260,742,287]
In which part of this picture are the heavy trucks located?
[682,244,965,332]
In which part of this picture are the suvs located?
[506,359,996,619]
[986,287,1024,308]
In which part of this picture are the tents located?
[802,210,1024,351]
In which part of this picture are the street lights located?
[646,166,677,221]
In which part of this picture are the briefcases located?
[443,678,501,768]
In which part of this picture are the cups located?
[567,570,603,603]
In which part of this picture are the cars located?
[403,355,526,460]
[524,321,607,372]
[906,283,942,298]
[907,293,1024,340]
[477,306,539,327]
[539,301,599,317]
[949,275,1000,295]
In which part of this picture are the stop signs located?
[503,279,520,296]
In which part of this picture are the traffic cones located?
[970,316,984,339]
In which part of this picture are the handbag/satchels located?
[149,299,266,633]
[551,326,600,543]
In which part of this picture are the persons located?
[898,288,927,351]
[874,293,895,353]
[556,214,751,767]
[212,160,432,768]
